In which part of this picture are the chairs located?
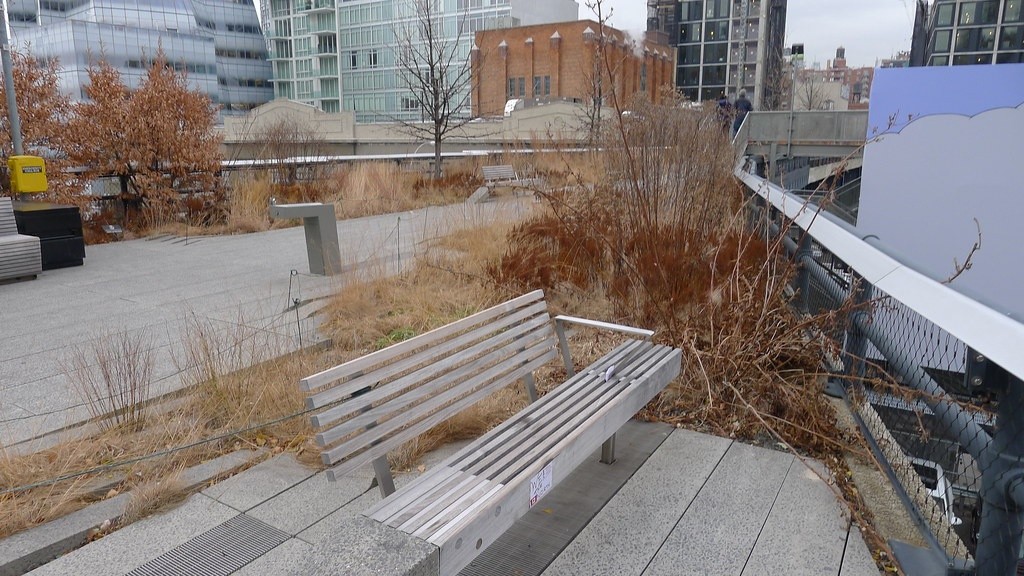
[0,195,42,283]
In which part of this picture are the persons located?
[717,93,732,141]
[733,88,753,136]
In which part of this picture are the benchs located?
[481,165,525,195]
[296,290,688,575]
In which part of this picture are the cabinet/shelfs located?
[14,199,87,269]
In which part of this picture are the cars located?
[905,455,964,530]
